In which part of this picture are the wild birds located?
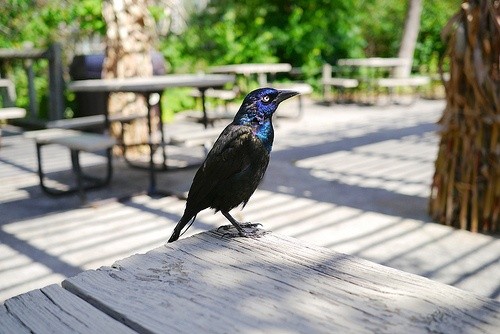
[168,88,300,242]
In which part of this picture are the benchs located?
[25,114,150,194]
[0,108,27,132]
[182,83,314,121]
[320,76,428,106]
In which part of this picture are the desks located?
[339,55,406,104]
[208,63,291,129]
[75,74,235,195]
[0,222,500,333]
